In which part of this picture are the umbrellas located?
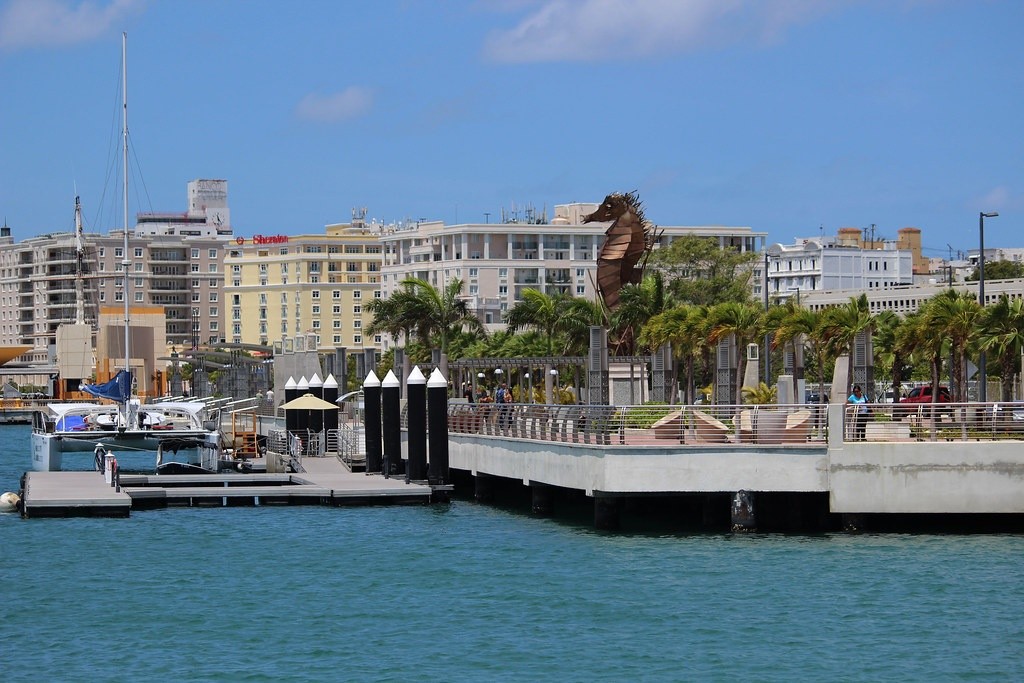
[277,393,340,431]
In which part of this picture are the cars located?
[979,399,1024,431]
[875,389,894,404]
[806,392,829,408]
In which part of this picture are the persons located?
[265,387,275,399]
[845,386,874,441]
[255,389,263,398]
[466,383,513,430]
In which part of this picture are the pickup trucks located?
[900,386,950,418]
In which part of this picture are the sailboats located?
[44,27,214,453]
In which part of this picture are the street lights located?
[979,212,998,419]
[764,252,781,389]
[789,287,799,308]
[939,264,956,403]
